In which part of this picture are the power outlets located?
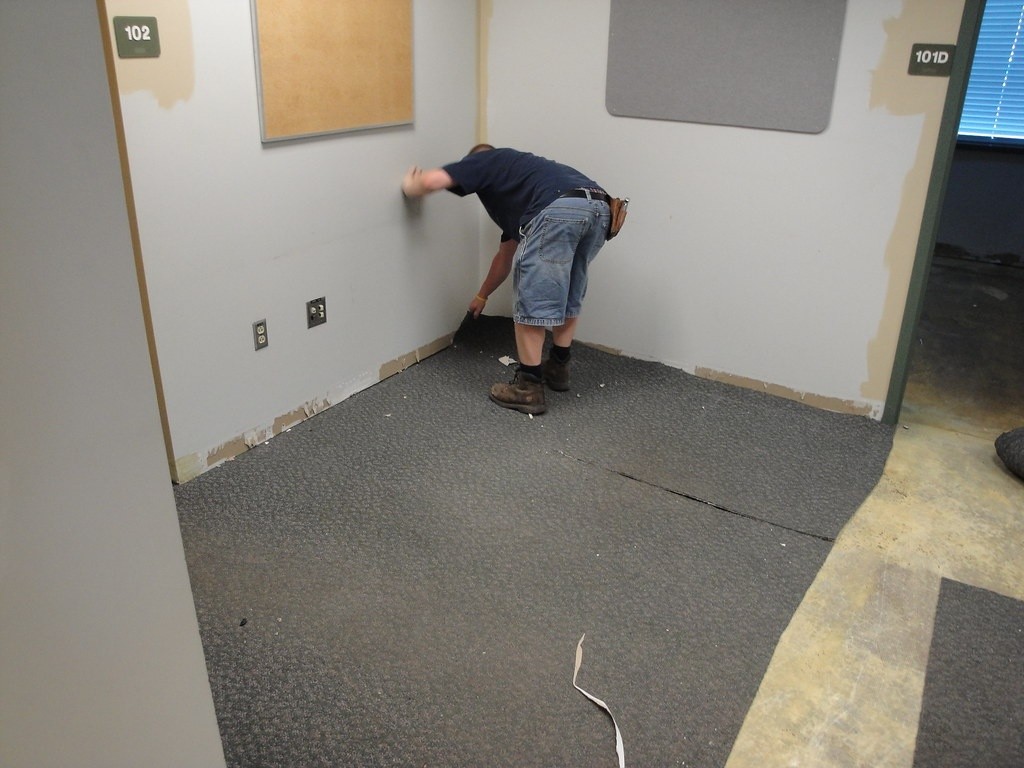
[305,296,328,329]
[251,318,269,350]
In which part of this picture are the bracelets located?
[475,295,488,301]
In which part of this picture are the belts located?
[560,190,611,204]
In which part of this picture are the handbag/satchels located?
[607,197,629,241]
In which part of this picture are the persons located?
[403,143,611,413]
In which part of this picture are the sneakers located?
[542,360,570,391]
[490,366,545,415]
